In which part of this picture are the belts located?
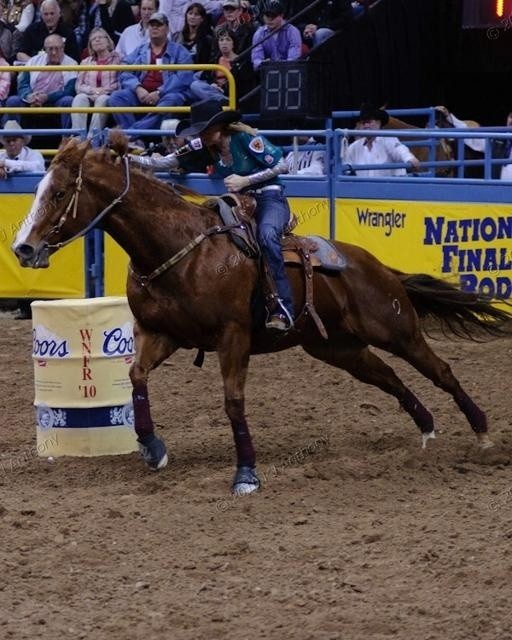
[251,185,281,194]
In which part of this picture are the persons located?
[1,1,511,183]
[114,97,294,333]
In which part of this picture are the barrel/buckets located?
[30,296,138,457]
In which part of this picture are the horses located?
[11,128,511,499]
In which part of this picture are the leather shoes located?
[265,314,287,331]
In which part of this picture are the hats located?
[0,120,31,146]
[148,13,169,27]
[222,0,240,7]
[352,104,389,125]
[262,0,281,16]
[176,96,240,137]
